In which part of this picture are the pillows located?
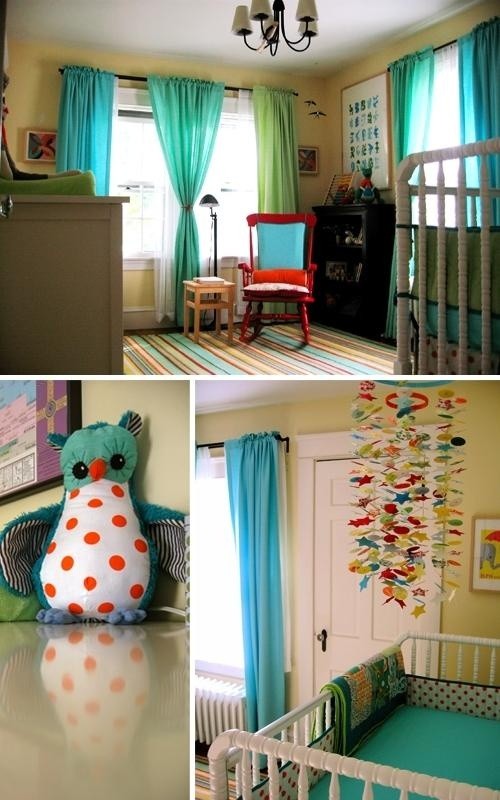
[250,269,308,284]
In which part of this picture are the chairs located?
[235,213,320,341]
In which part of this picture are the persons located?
[0,410,189,625]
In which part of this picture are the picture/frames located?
[296,145,320,175]
[470,514,500,595]
[338,68,397,193]
[1,380,78,503]
[22,127,59,165]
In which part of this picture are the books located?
[193,277,224,284]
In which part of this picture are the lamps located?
[232,1,319,54]
[200,195,221,329]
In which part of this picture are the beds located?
[204,630,500,800]
[183,281,235,343]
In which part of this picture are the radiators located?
[193,675,248,751]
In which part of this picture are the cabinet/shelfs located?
[309,205,388,335]
